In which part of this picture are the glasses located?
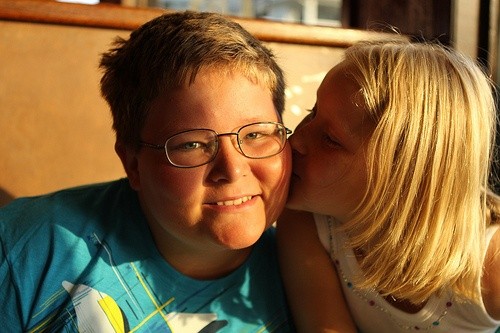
[131,122,292,168]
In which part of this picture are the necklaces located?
[325,216,457,332]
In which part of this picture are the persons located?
[0,11,293,333]
[273,38,500,333]
